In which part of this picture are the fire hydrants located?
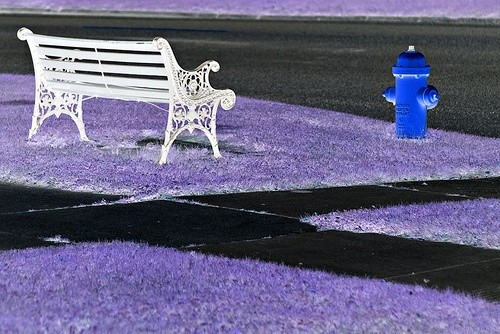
[382,45,440,138]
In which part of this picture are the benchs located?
[17,28,238,165]
